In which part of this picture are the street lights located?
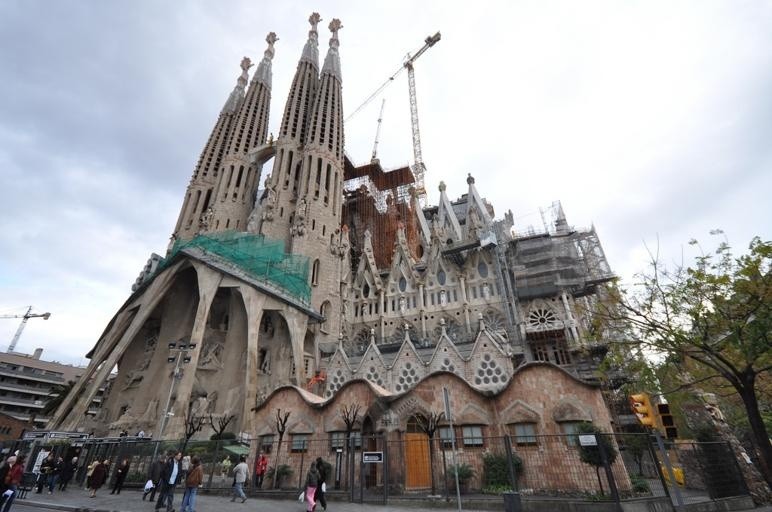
[152,343,196,459]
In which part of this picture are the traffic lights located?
[630,392,658,431]
[656,403,679,441]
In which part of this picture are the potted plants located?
[444,463,478,493]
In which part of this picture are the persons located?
[256,451,267,488]
[220,453,232,484]
[230,456,250,503]
[0,449,205,512]
[300,460,323,512]
[313,456,328,511]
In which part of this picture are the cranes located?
[346,30,441,207]
[0,307,50,352]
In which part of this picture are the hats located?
[7,456,16,462]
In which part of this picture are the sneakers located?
[143,494,175,512]
[230,499,235,502]
[241,497,247,503]
[306,503,317,512]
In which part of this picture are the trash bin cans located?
[503,492,522,512]
